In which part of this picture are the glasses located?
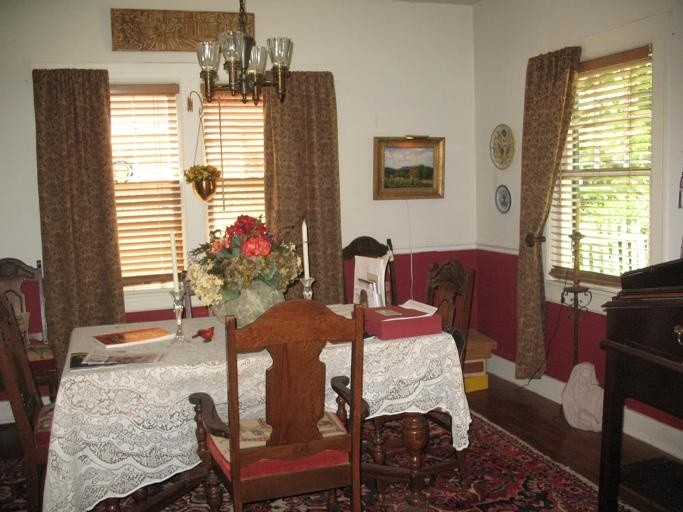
[196,0,293,106]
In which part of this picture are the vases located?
[183,164,221,184]
[184,214,303,306]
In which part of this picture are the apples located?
[302,219,309,279]
[170,231,179,293]
[576,202,580,231]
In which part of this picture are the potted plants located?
[494,184,512,214]
[372,134,444,200]
[211,281,286,329]
[489,123,515,170]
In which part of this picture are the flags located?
[194,177,216,203]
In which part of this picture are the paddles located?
[0,410,636,511]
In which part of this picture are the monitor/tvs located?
[93,326,172,349]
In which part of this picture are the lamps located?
[343,235,398,308]
[0,293,149,512]
[188,299,365,512]
[371,256,476,492]
[0,258,58,402]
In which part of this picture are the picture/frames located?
[41,304,473,512]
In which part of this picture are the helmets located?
[489,124,516,169]
[495,185,511,214]
